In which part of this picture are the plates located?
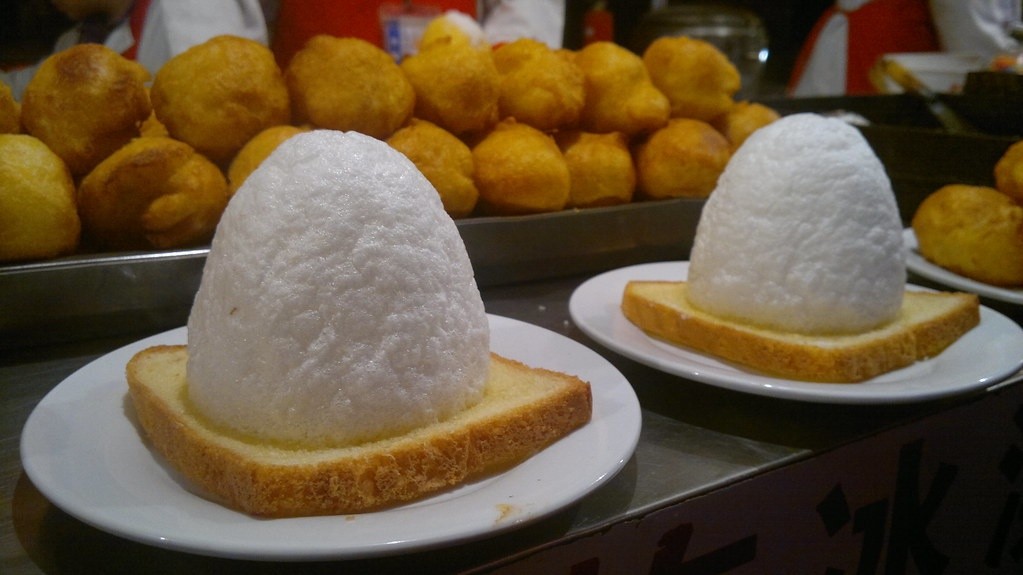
[17,311,643,562]
[897,228,1023,307]
[566,260,1023,404]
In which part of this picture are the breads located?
[619,278,981,385]
[126,338,594,518]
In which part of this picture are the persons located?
[0,1,1023,103]
